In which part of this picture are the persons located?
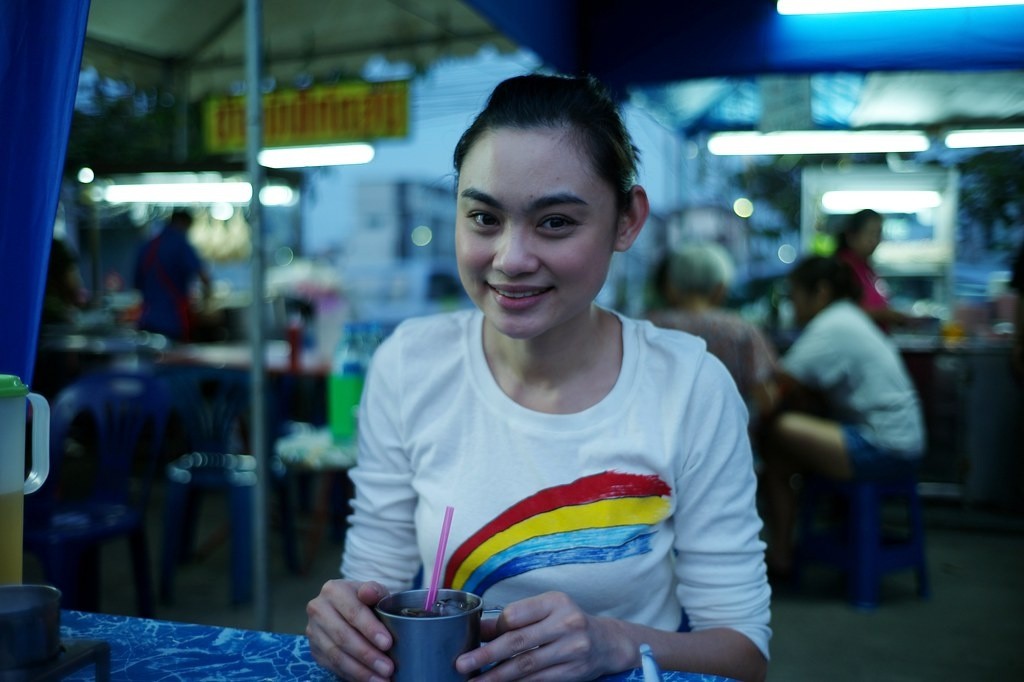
[836,210,943,336]
[762,255,923,569]
[306,76,773,682]
[131,207,211,342]
[45,239,88,309]
[646,244,780,409]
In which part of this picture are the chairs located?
[27,365,170,617]
[150,365,302,605]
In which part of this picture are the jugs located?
[1,373,51,586]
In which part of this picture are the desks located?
[57,611,736,682]
[280,423,355,578]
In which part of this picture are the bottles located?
[325,316,387,450]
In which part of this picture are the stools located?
[791,473,931,608]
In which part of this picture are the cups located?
[373,588,505,682]
[0,583,63,669]
